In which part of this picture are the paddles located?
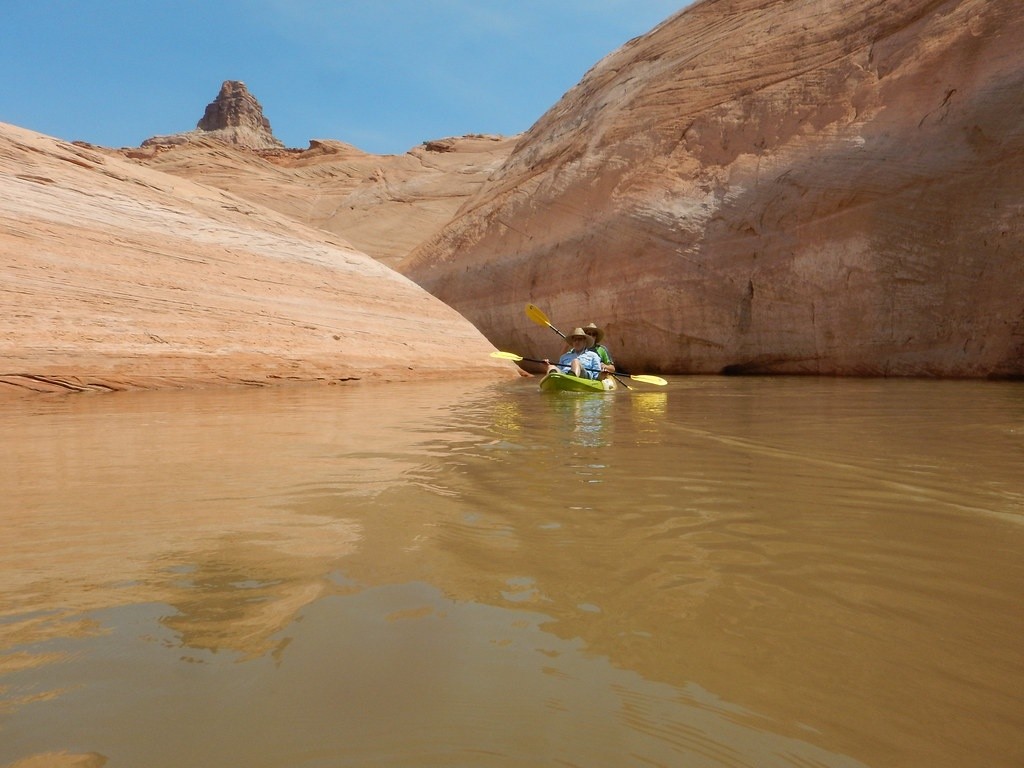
[490,351,668,386]
[525,303,633,391]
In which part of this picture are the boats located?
[539,372,618,393]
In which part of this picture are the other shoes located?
[549,369,557,373]
[567,371,576,376]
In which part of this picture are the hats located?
[566,328,594,348]
[582,323,604,343]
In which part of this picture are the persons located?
[561,323,615,381]
[539,328,609,381]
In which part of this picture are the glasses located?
[571,338,584,341]
[585,332,596,336]
